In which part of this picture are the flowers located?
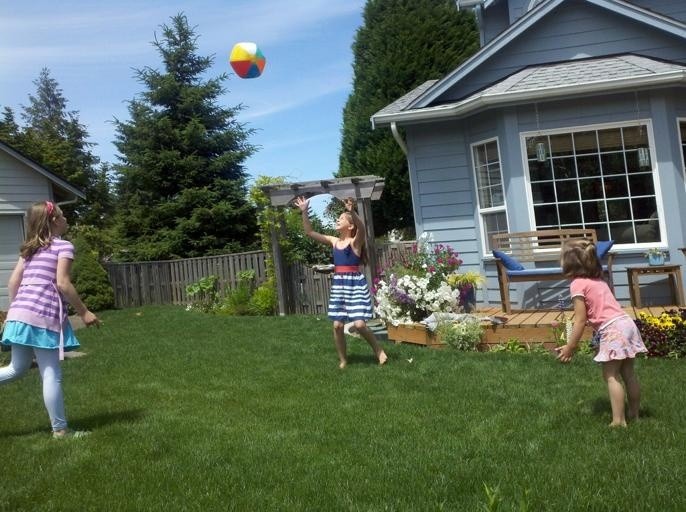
[370,232,472,327]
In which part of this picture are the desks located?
[625,263,685,309]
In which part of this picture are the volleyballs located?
[229,42,265,78]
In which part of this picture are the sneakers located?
[53,430,90,439]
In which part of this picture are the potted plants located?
[644,247,667,265]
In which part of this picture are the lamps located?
[535,99,546,163]
[634,89,649,167]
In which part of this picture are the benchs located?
[492,229,615,315]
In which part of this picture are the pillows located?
[595,239,615,259]
[492,249,524,270]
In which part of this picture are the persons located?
[0,199,104,440]
[291,192,389,369]
[552,236,650,429]
